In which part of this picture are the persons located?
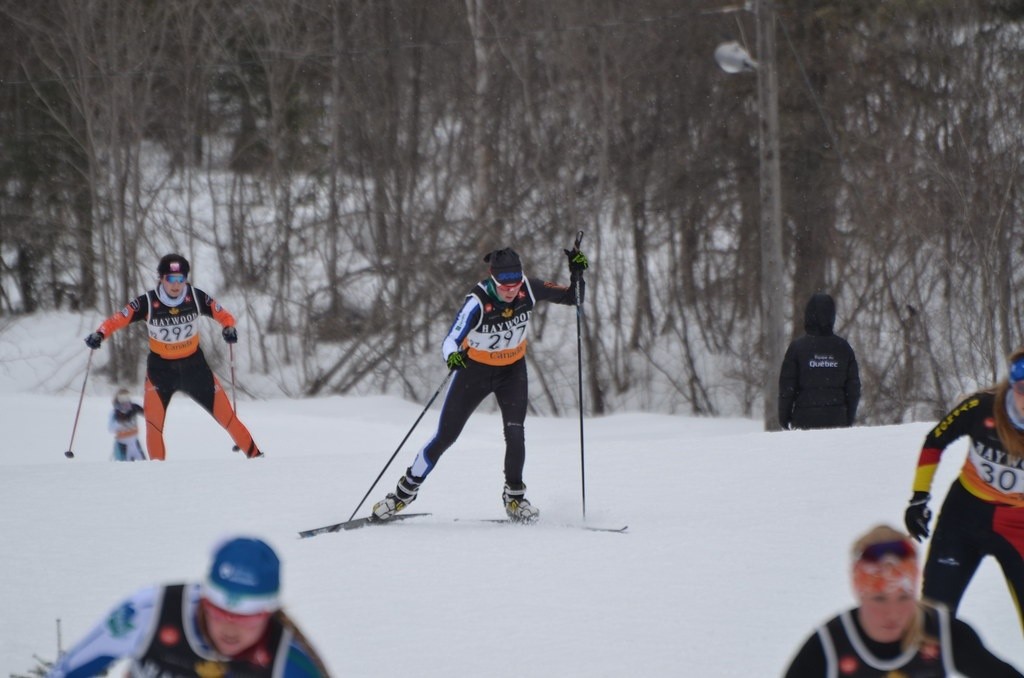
[84,254,263,461]
[904,342,1024,636]
[372,248,589,522]
[108,389,145,461]
[778,294,862,431]
[782,524,1024,678]
[44,536,331,678]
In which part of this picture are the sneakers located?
[372,477,419,520]
[505,483,540,521]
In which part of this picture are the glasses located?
[1012,382,1024,395]
[491,274,524,291]
[201,598,266,622]
[854,541,916,572]
[162,275,185,283]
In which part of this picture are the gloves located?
[564,248,588,277]
[84,332,103,349]
[446,352,468,371]
[905,490,931,542]
[222,327,237,342]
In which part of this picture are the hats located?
[200,539,283,614]
[853,525,921,594]
[157,254,190,275]
[483,247,523,284]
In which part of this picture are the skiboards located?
[292,512,630,535]
